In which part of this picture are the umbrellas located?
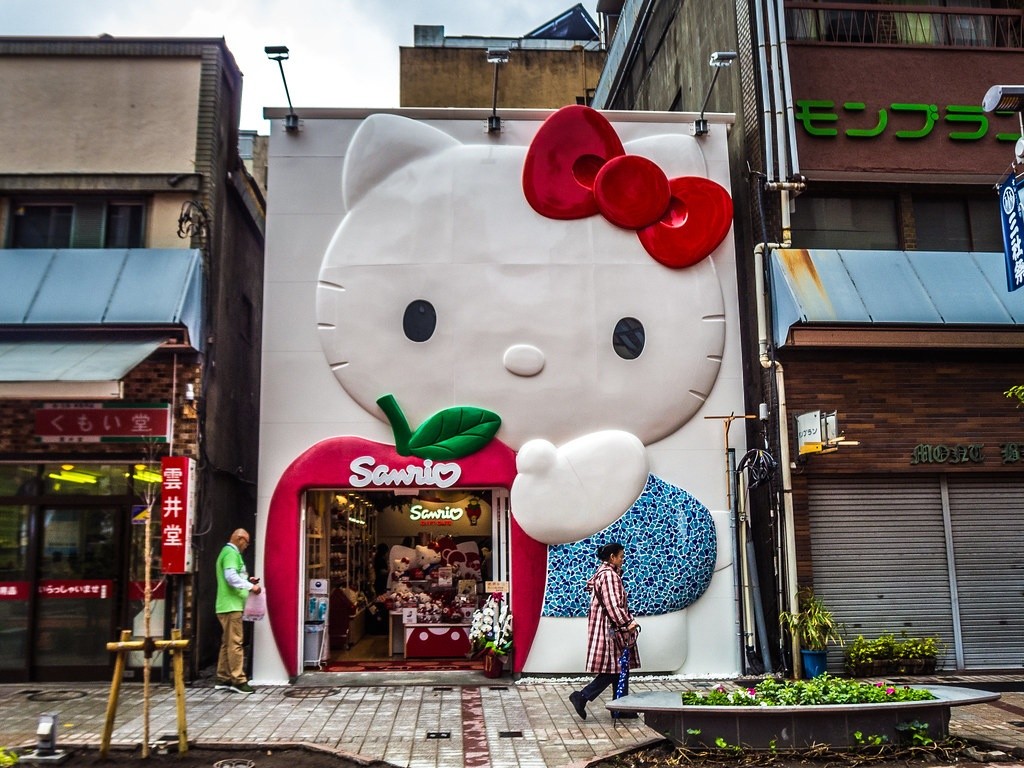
[613,624,642,728]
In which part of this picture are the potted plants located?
[848,635,899,677]
[781,587,844,682]
[898,635,946,675]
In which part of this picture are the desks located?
[389,610,444,657]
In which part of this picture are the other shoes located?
[611,711,639,719]
[229,681,255,694]
[215,679,230,689]
[569,691,588,720]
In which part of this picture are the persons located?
[214,528,262,694]
[568,542,638,720]
[67,551,95,579]
[373,542,389,612]
[51,552,72,572]
[402,537,412,548]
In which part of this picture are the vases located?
[484,656,503,680]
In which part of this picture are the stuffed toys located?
[415,545,441,580]
[393,557,410,581]
[333,499,368,610]
[387,581,465,623]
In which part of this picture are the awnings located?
[0,241,204,401]
[769,249,1024,349]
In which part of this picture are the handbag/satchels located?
[614,625,639,649]
[242,588,266,621]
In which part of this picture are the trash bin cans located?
[304,620,331,666]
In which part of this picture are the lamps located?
[484,46,511,131]
[694,52,739,133]
[264,45,302,129]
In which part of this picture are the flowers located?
[683,674,933,705]
[468,592,514,659]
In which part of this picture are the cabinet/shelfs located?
[302,487,380,646]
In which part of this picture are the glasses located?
[239,536,250,546]
